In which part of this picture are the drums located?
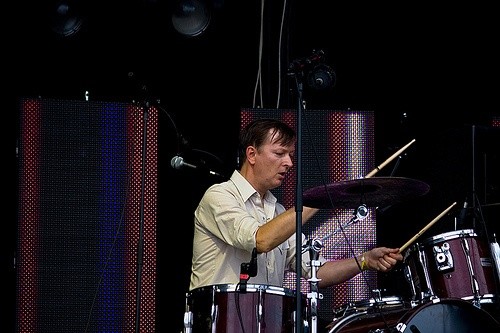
[182,282,311,333]
[403,228,500,307]
[332,295,402,317]
[322,295,500,333]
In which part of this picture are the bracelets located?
[361,252,367,269]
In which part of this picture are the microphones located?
[288,50,323,72]
[247,248,260,277]
[169,155,220,176]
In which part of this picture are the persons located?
[188,119,403,288]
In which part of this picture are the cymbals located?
[302,176,430,209]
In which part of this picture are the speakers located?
[15,97,156,333]
[238,105,378,331]
